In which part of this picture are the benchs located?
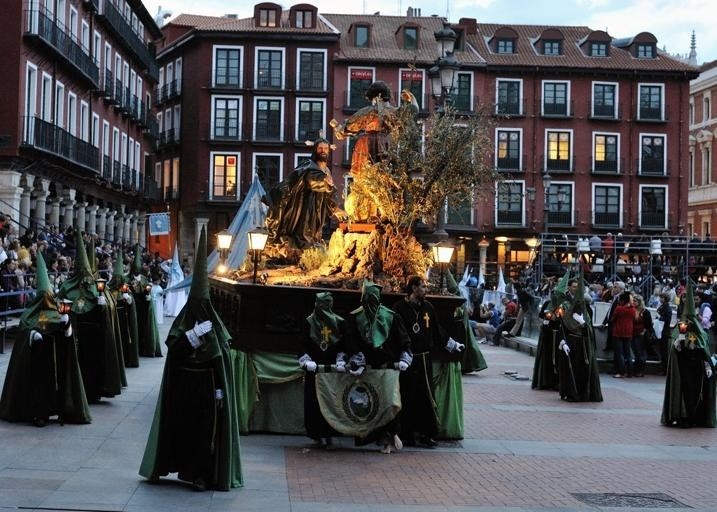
[474,287,542,357]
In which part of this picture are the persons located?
[137,225,488,492]
[474,226,716,427]
[330,81,420,220]
[0,212,189,426]
[267,139,340,267]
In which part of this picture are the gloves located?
[307,361,317,371]
[123,293,129,299]
[34,332,42,340]
[399,361,409,371]
[563,344,571,356]
[456,342,465,352]
[705,367,713,378]
[194,320,213,337]
[98,296,106,305]
[573,313,586,326]
[60,314,69,324]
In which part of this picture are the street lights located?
[422,15,466,243]
[527,173,567,234]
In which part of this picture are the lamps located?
[432,238,457,292]
[212,227,233,275]
[246,225,269,283]
